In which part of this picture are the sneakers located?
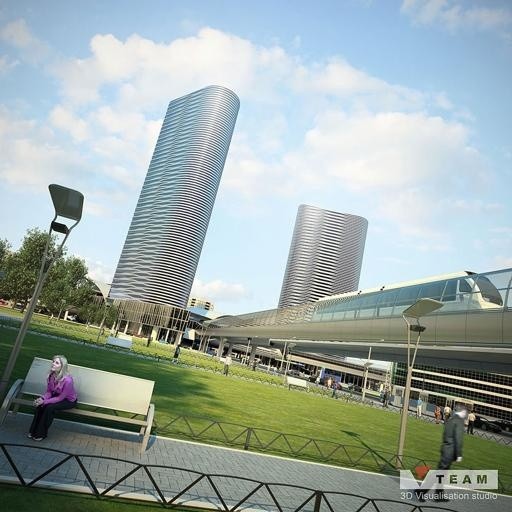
[24,432,47,442]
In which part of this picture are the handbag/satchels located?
[51,390,59,397]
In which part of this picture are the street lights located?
[395,298,444,472]
[1,184,84,403]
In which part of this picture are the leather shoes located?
[414,489,425,502]
[432,495,450,502]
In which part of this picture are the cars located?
[489,418,511,432]
[474,415,501,433]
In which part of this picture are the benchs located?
[118,335,132,341]
[0,357,156,451]
[286,376,309,392]
[106,336,132,352]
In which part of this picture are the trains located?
[202,270,503,327]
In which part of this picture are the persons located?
[171,345,180,365]
[380,385,392,409]
[222,354,232,376]
[24,354,78,442]
[314,375,360,398]
[413,406,468,502]
[416,397,481,436]
[251,359,258,371]
